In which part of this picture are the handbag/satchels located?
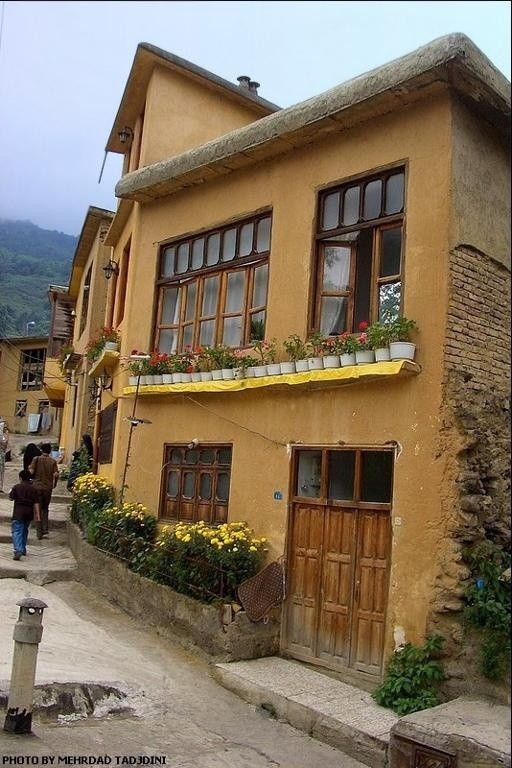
[28,469,36,479]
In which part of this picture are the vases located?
[62,341,416,385]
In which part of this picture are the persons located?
[7,469,43,562]
[23,442,43,480]
[1,415,11,495]
[66,434,94,493]
[27,442,59,540]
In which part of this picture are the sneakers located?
[13,550,26,560]
[37,528,48,538]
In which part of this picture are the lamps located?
[118,126,134,144]
[103,258,120,279]
[187,438,199,450]
[88,372,113,400]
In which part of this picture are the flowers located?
[56,310,422,375]
